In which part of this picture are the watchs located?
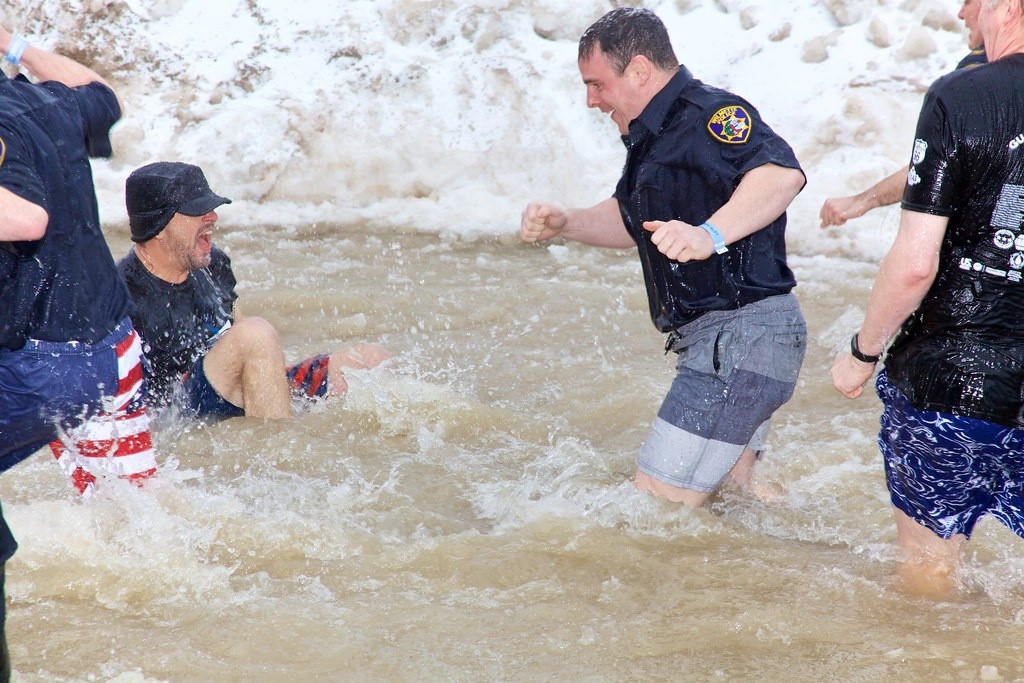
[850,334,884,363]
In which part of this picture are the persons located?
[0,24,136,679]
[518,3,809,520]
[115,159,387,419]
[817,0,988,231]
[831,1,1024,602]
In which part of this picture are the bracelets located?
[698,222,728,254]
[5,32,28,63]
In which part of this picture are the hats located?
[126,161,232,243]
